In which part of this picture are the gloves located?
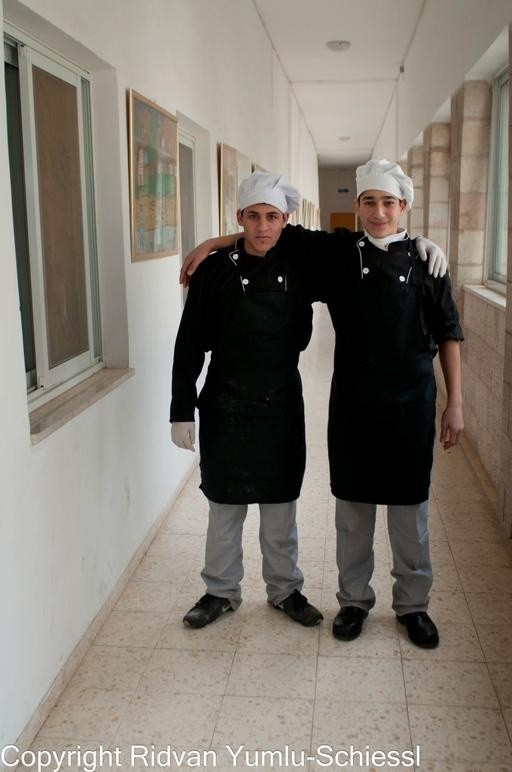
[171,422,195,452]
[416,236,447,278]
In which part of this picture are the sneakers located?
[273,589,323,626]
[183,594,232,629]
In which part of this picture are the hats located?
[356,159,414,209]
[237,174,301,213]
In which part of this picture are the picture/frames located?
[124,86,182,265]
[218,140,240,238]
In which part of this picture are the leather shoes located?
[332,606,368,641]
[396,612,439,648]
